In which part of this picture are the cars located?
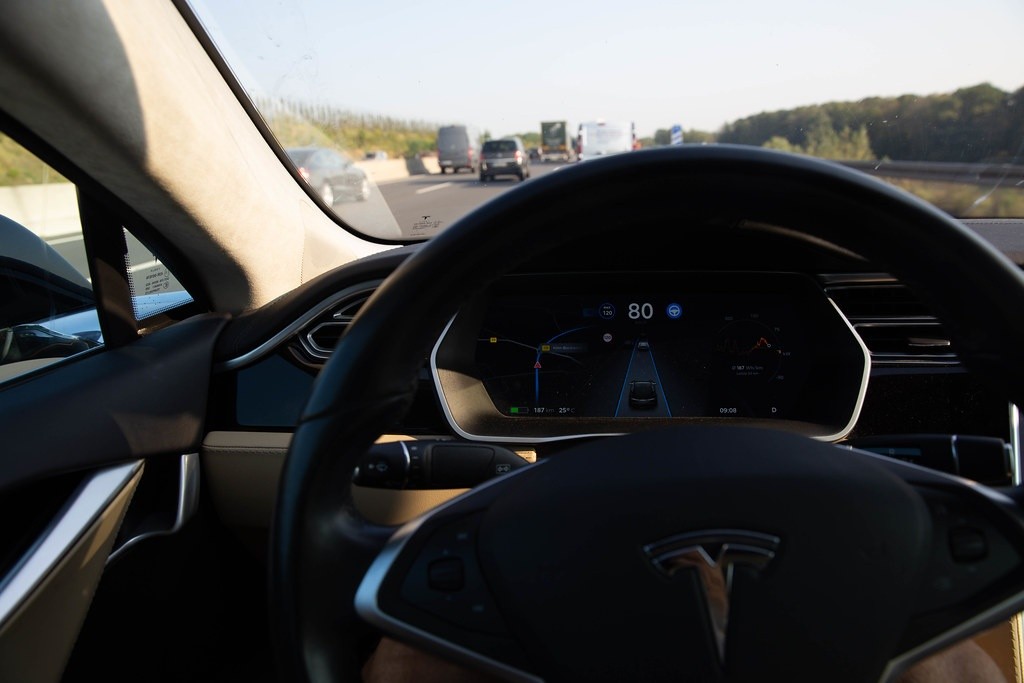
[280,146,371,210]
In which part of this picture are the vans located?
[437,123,480,175]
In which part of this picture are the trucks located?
[540,120,571,161]
[579,118,637,164]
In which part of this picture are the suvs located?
[479,137,533,180]
